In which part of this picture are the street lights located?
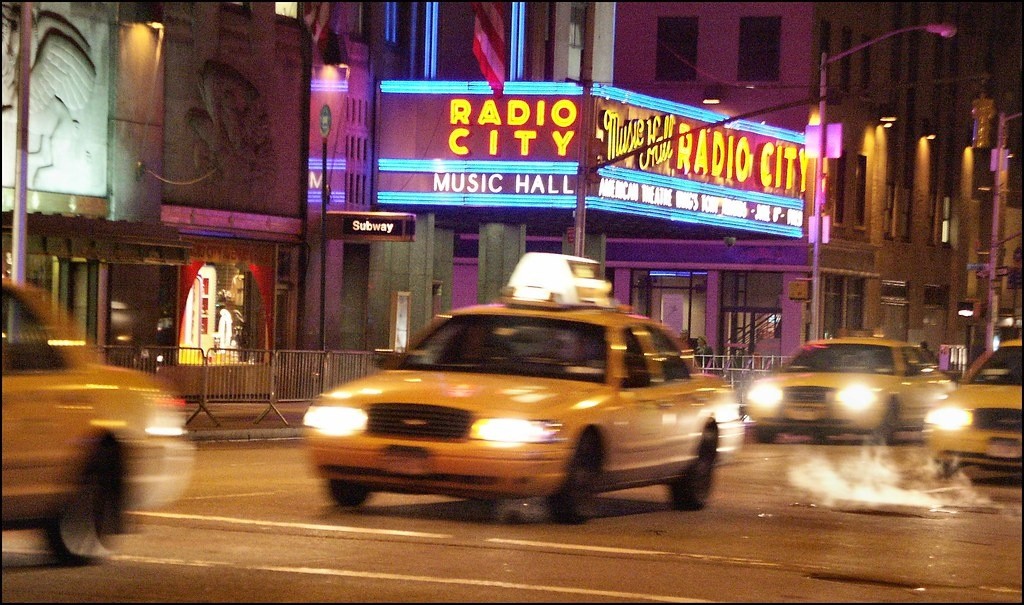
[806,19,957,344]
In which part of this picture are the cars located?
[3,279,192,563]
[924,335,1023,486]
[751,335,958,451]
[299,250,739,520]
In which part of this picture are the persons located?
[918,342,939,369]
[441,323,586,379]
[694,335,715,376]
[676,329,694,355]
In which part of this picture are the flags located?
[471,0,513,99]
[300,0,344,65]
